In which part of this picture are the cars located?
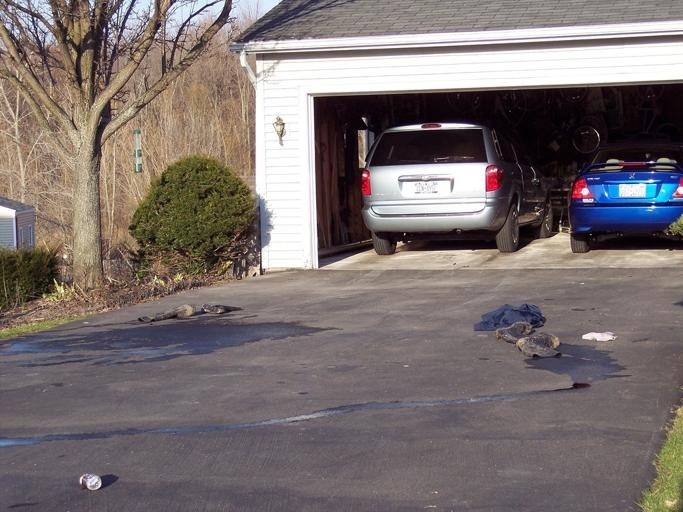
[568,142,683,253]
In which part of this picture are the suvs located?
[361,122,553,256]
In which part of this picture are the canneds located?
[80,474,102,491]
